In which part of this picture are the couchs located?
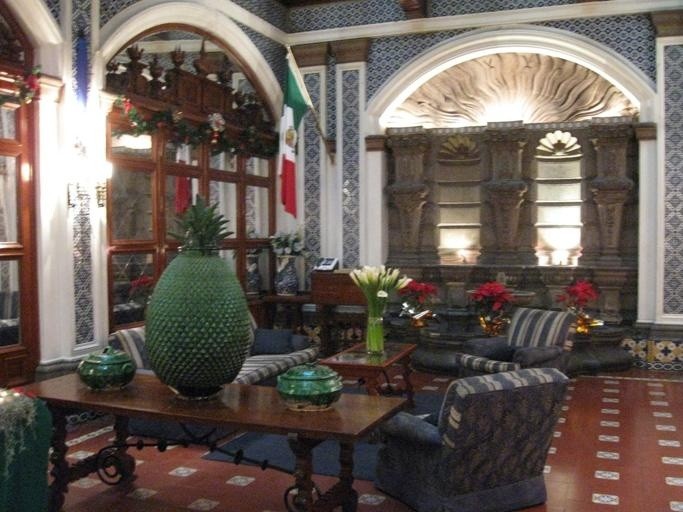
[109,309,320,444]
[455,308,575,379]
[377,368,567,509]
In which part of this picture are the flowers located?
[557,280,599,314]
[349,264,412,316]
[399,280,437,311]
[470,282,515,316]
[114,95,280,158]
[267,231,306,256]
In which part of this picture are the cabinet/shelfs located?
[310,267,369,352]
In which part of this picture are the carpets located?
[202,387,445,482]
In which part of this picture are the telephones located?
[314,257,340,273]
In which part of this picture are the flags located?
[278,55,313,218]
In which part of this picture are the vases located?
[277,256,297,295]
[577,317,590,333]
[480,318,508,336]
[409,312,430,326]
[367,317,385,354]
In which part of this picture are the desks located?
[7,370,407,511]
